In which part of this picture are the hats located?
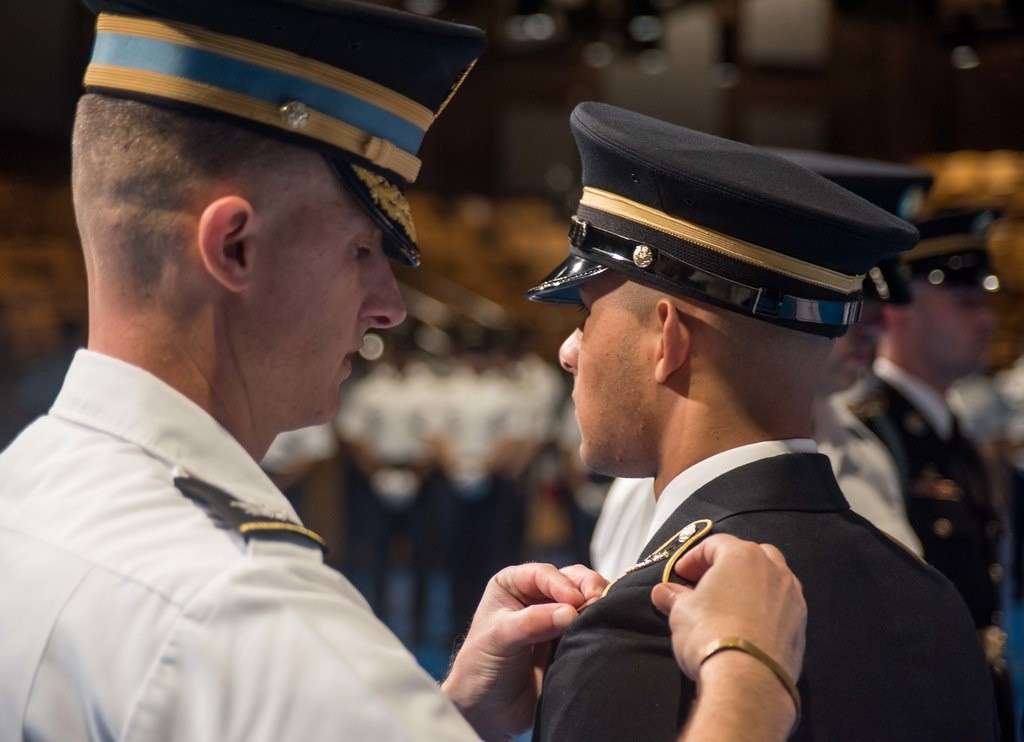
[527,100,922,339]
[85,0,485,270]
[898,203,1009,301]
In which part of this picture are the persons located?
[271,338,1024,684]
[832,201,1023,738]
[590,146,922,584]
[524,101,1018,742]
[1,2,808,742]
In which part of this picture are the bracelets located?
[695,636,802,736]
